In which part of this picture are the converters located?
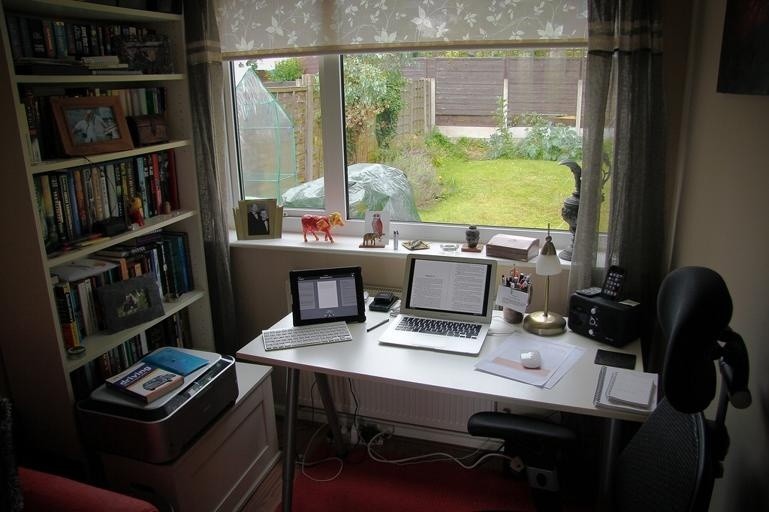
[350,424,359,445]
[360,427,380,443]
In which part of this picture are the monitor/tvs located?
[288,266,366,327]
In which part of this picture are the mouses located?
[521,349,542,369]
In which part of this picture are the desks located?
[235,296,654,511]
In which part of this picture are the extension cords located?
[344,435,385,447]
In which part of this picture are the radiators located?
[292,369,505,453]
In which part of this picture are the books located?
[50,228,195,352]
[69,353,142,403]
[605,370,654,409]
[105,346,211,404]
[32,149,181,257]
[4,9,180,74]
[593,365,659,416]
[18,84,174,166]
[145,306,194,353]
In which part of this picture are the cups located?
[503,285,533,324]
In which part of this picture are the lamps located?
[523,223,568,336]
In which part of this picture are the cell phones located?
[370,296,398,312]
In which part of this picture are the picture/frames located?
[233,199,283,239]
[49,96,133,154]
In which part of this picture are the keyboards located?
[261,320,353,352]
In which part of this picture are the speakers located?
[567,291,643,348]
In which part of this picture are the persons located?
[257,209,270,234]
[248,203,260,234]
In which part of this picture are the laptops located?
[379,254,499,357]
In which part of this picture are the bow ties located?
[263,219,268,222]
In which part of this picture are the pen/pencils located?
[502,264,532,290]
[366,319,390,332]
[64,235,112,253]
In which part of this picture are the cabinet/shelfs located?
[0,1,218,477]
[94,362,284,512]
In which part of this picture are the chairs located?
[467,266,752,512]
